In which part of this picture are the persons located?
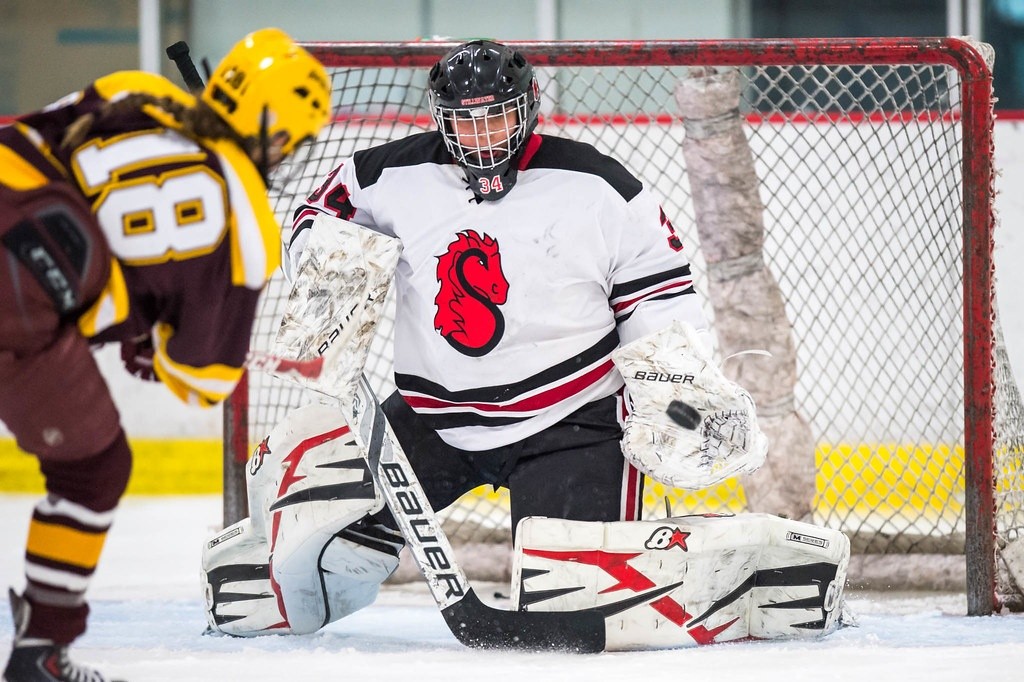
[0,28,334,682]
[200,40,851,654]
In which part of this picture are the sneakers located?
[0,587,107,682]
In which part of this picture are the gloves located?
[123,338,160,381]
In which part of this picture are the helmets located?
[428,40,539,200]
[200,27,333,153]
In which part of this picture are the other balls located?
[666,398,701,429]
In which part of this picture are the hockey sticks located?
[164,38,606,655]
